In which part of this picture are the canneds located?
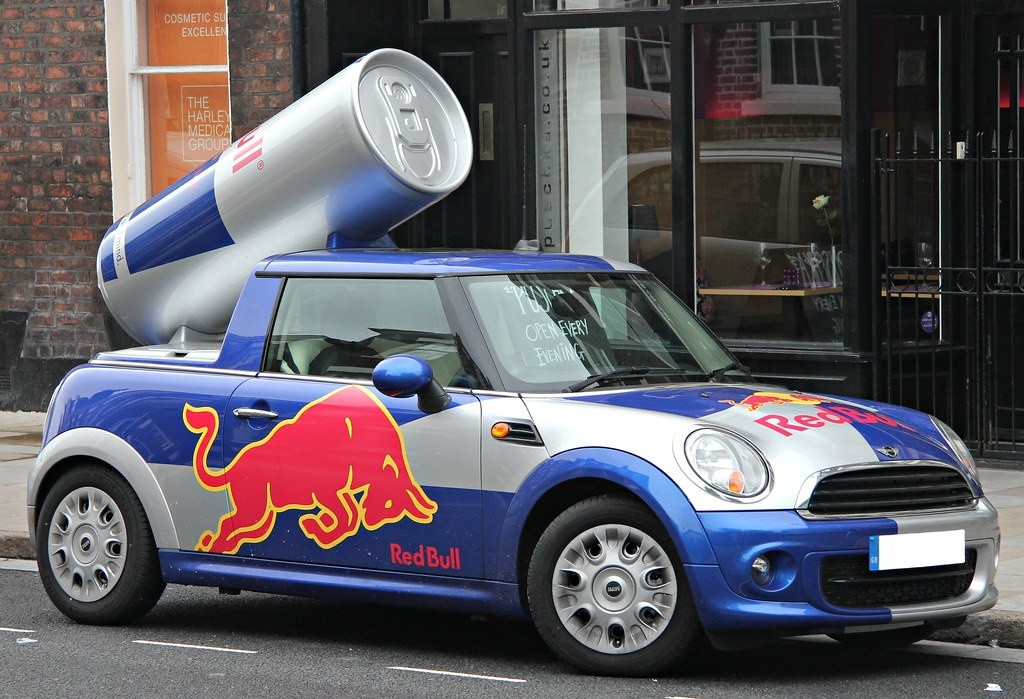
[96,47,473,345]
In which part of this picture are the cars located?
[24,47,1001,675]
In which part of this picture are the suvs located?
[571,144,844,329]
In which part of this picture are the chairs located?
[309,294,384,378]
